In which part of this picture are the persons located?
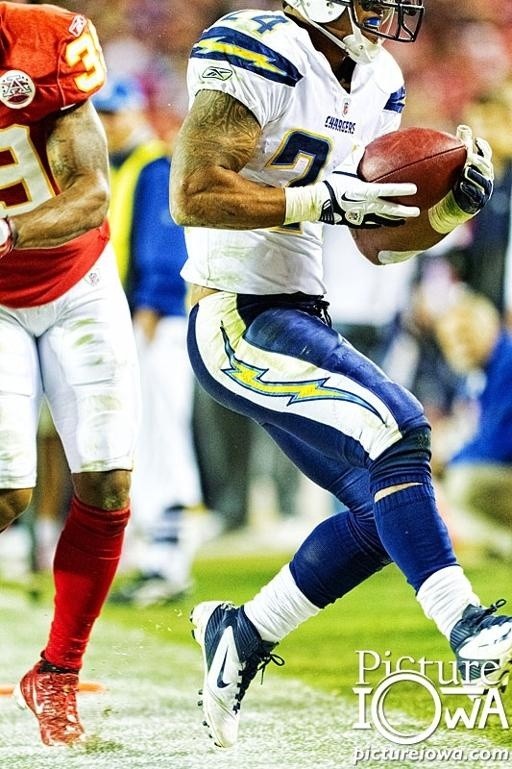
[0,1,512,752]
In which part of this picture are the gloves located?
[0,216,18,260]
[281,144,420,229]
[428,126,494,235]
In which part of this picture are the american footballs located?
[358,129,467,211]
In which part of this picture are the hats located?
[90,74,150,113]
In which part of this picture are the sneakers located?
[131,535,195,613]
[448,597,510,702]
[21,661,84,753]
[189,599,277,747]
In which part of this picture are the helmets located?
[286,0,425,66]
